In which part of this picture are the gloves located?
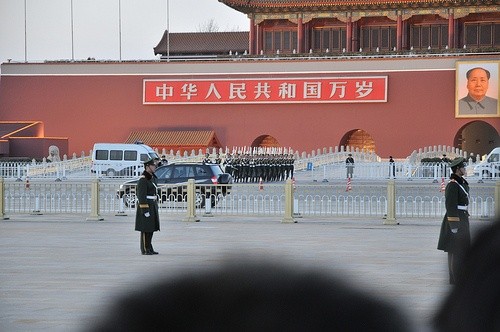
[451,227,457,233]
[144,212,150,218]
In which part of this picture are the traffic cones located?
[292,176,296,189]
[440,177,446,192]
[346,178,352,190]
[258,177,264,191]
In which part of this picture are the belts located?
[146,195,160,201]
[457,206,468,209]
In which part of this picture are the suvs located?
[116,161,234,210]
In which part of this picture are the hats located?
[348,154,352,156]
[204,153,293,156]
[449,157,464,167]
[144,158,155,165]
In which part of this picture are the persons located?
[153,155,168,168]
[135,158,161,255]
[222,153,295,184]
[436,157,473,284]
[202,152,213,163]
[387,155,397,179]
[345,153,355,179]
[214,152,221,164]
[457,67,498,115]
[441,153,449,180]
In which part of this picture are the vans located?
[473,147,500,177]
[91,141,164,179]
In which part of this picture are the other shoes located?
[141,251,158,255]
